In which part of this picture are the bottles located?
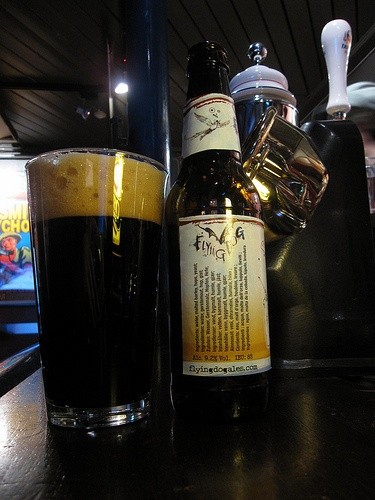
[165,39,280,435]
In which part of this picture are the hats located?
[0,231,22,243]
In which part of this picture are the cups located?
[24,145,166,442]
